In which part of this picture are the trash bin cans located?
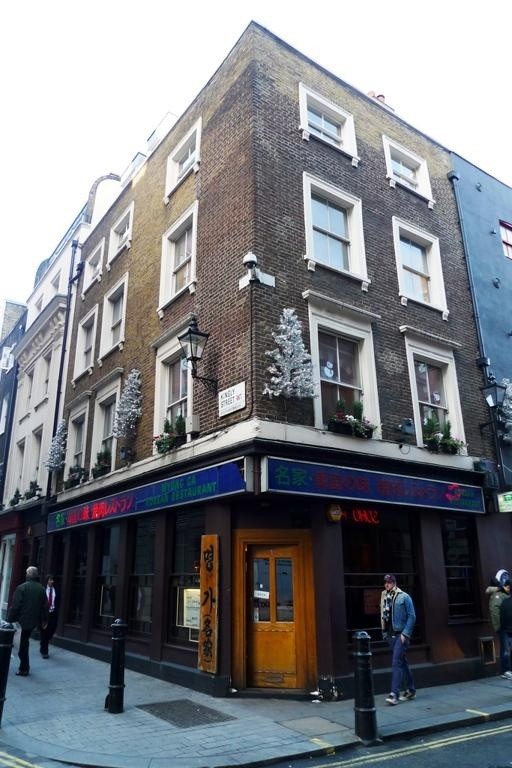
[0,623,17,724]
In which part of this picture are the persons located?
[6,566,49,675]
[499,572,512,680]
[40,574,59,659]
[380,575,416,705]
[485,569,509,679]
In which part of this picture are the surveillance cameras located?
[243,254,258,270]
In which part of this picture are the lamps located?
[176,315,220,395]
[475,369,508,435]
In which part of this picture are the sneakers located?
[400,689,416,702]
[16,670,29,676]
[384,690,400,705]
[40,648,49,659]
[499,670,512,680]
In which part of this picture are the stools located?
[477,636,496,666]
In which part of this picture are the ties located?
[47,586,54,612]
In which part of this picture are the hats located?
[382,572,396,584]
